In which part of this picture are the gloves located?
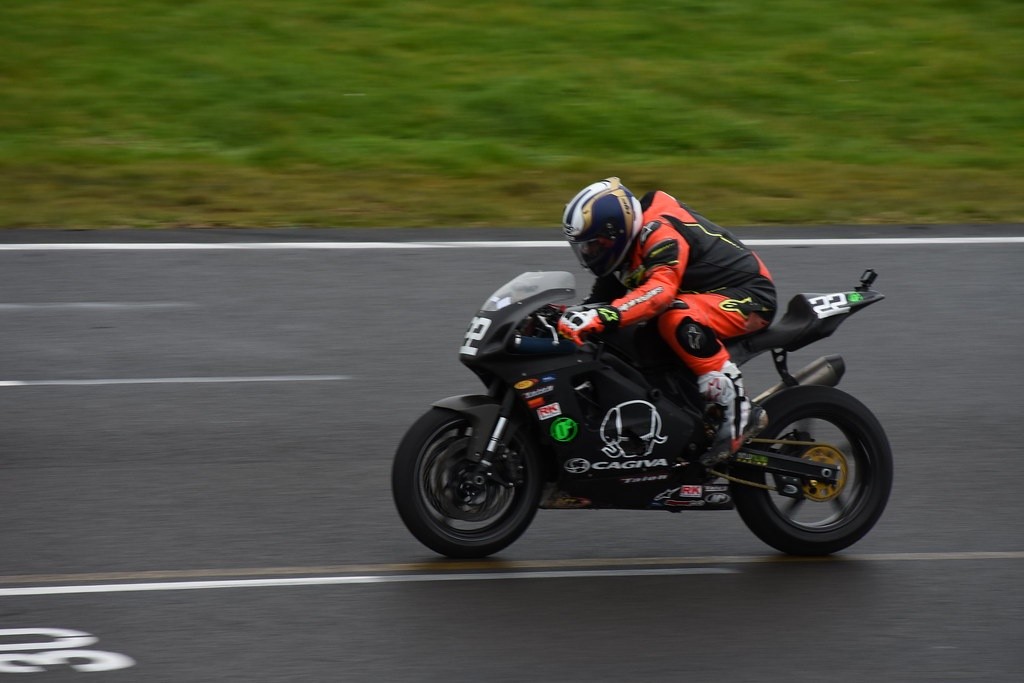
[559,301,620,346]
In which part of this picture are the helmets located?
[560,176,642,279]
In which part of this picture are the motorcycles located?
[392,268,897,561]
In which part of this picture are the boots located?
[696,359,769,464]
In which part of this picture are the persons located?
[557,176,777,467]
